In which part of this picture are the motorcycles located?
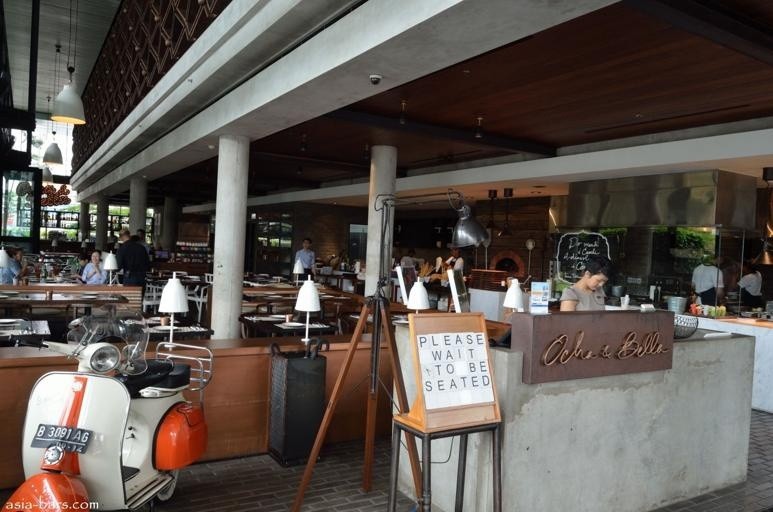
[0,302,213,512]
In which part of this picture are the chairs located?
[272,276,283,283]
[173,271,188,280]
[143,279,162,315]
[186,284,209,324]
[243,281,255,287]
[201,273,214,284]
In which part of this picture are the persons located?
[0,245,35,285]
[71,253,89,284]
[116,228,152,313]
[82,249,107,284]
[446,248,463,276]
[408,248,416,258]
[12,247,40,277]
[131,235,141,244]
[690,250,724,306]
[559,255,613,311]
[136,229,150,255]
[447,247,455,266]
[295,237,317,282]
[732,258,766,311]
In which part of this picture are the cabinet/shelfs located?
[0,333,394,490]
[605,304,773,415]
[388,320,756,512]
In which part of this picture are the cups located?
[13,279,18,285]
[161,318,168,326]
[23,279,28,285]
[620,296,630,308]
[48,271,53,278]
[286,315,294,322]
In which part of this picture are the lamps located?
[157,271,190,353]
[42,42,64,165]
[292,258,305,283]
[41,92,55,185]
[503,277,525,314]
[293,274,322,347]
[49,0,88,127]
[291,188,491,512]
[406,274,431,315]
[100,250,119,287]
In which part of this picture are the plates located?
[153,325,178,330]
[282,322,305,326]
[151,318,161,320]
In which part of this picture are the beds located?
[0,290,129,320]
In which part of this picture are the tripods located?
[292,200,425,511]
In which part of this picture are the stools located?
[272,323,339,337]
[238,312,297,338]
[118,315,181,329]
[388,413,504,512]
[348,311,412,334]
[140,326,215,342]
